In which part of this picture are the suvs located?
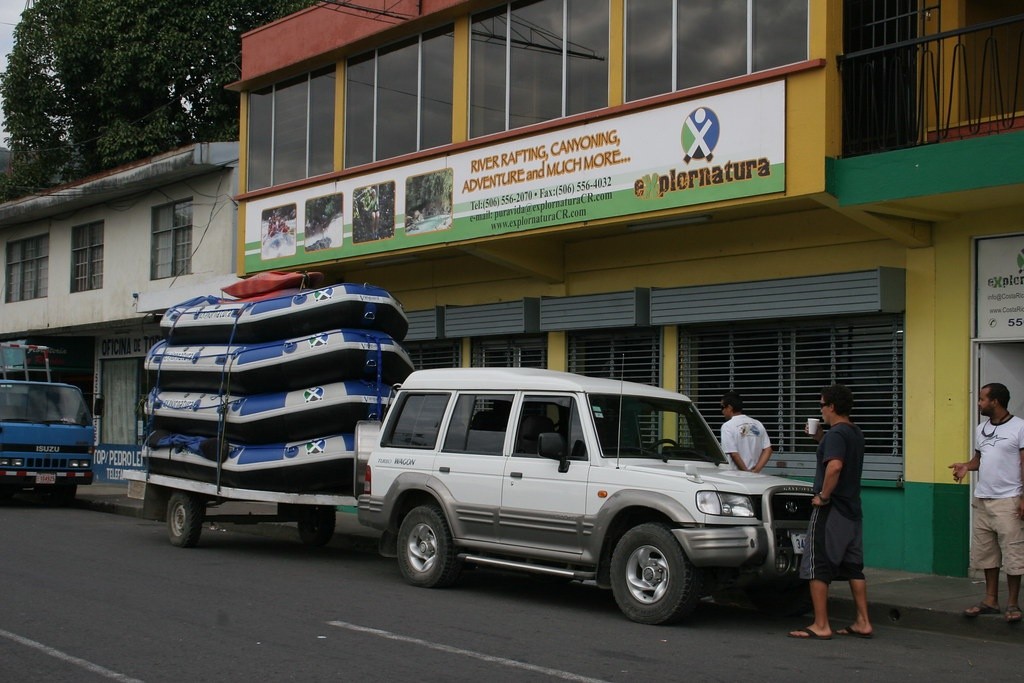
[356,366,814,627]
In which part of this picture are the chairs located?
[517,416,557,455]
[536,432,564,457]
[468,410,505,432]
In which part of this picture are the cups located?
[807,418,820,435]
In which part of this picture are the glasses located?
[721,405,729,410]
[820,402,833,409]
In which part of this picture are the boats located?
[142,337,416,398]
[141,377,402,446]
[141,427,363,496]
[159,279,409,348]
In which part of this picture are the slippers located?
[1005,604,1022,622]
[788,628,833,639]
[963,602,1001,615]
[836,623,872,638]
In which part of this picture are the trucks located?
[0,342,105,502]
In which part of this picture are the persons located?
[721,392,773,473]
[786,385,873,638]
[948,383,1024,619]
[268,213,290,237]
[364,184,380,238]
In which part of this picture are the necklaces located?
[982,412,1009,437]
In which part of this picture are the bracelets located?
[819,496,829,502]
